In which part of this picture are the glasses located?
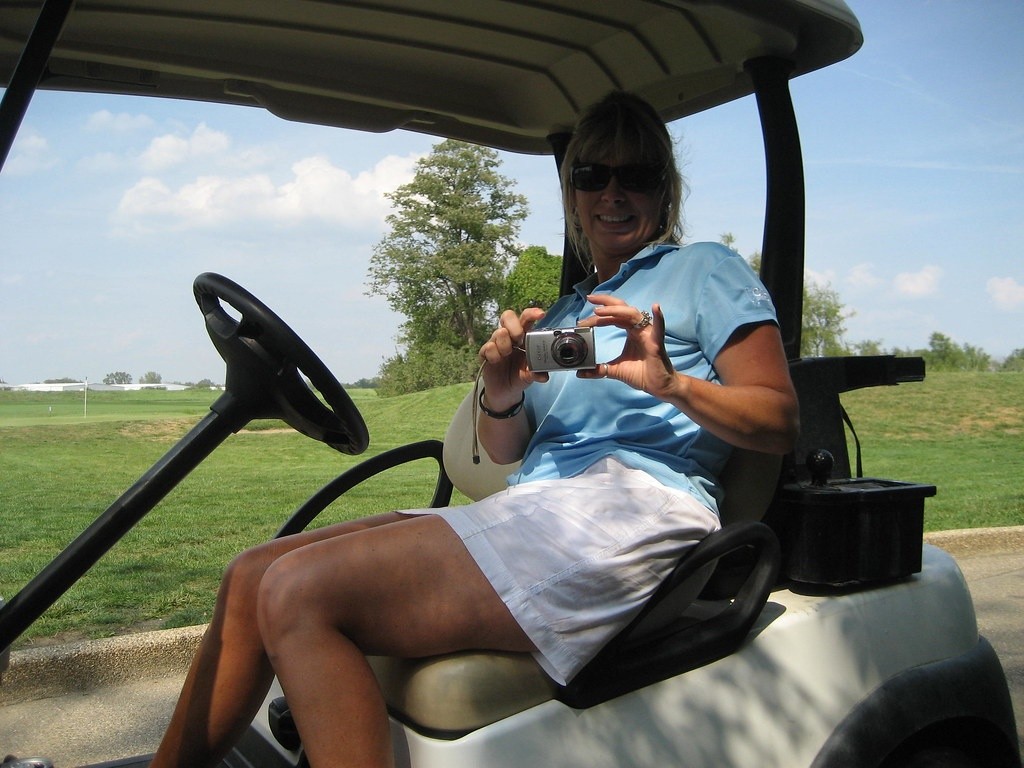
[569,159,668,193]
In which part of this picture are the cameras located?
[524,327,597,372]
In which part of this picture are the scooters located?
[4,0,1024,768]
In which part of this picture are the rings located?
[631,310,652,330]
[602,363,608,378]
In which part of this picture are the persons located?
[148,90,802,768]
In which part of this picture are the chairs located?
[366,432,787,737]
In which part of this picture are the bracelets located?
[478,385,526,419]
